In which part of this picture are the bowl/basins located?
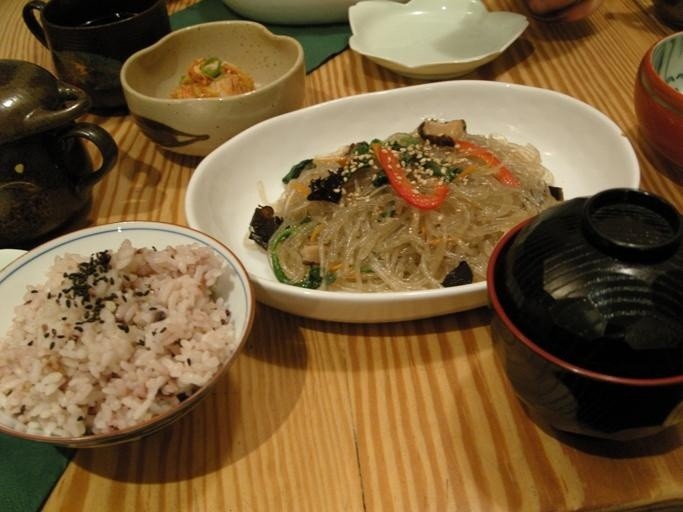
[0,219,254,452]
[489,188,683,462]
[121,20,306,160]
[636,29,683,173]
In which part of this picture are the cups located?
[19,0,171,115]
[0,59,119,245]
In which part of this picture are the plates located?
[345,1,528,78]
[185,86,639,324]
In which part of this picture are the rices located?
[0,237,235,438]
